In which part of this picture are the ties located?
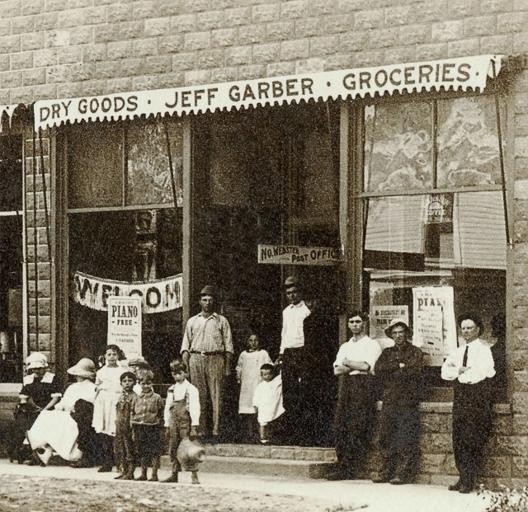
[463,346,468,369]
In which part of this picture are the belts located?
[190,349,224,355]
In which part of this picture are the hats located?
[200,285,215,296]
[176,437,206,470]
[285,276,299,285]
[128,358,150,369]
[25,352,48,371]
[457,311,485,336]
[383,318,413,338]
[67,358,96,379]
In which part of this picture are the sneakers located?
[449,477,465,491]
[70,458,94,468]
[324,466,349,480]
[350,467,367,480]
[98,463,113,472]
[390,471,413,484]
[372,469,392,482]
[460,477,474,492]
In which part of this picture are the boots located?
[114,474,200,484]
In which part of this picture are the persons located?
[5,352,63,463]
[324,309,383,481]
[116,372,139,479]
[279,276,312,445]
[179,286,234,440]
[128,354,150,465]
[374,317,424,483]
[252,359,286,443]
[21,357,98,465]
[441,313,497,493]
[92,345,129,472]
[133,370,164,480]
[163,359,206,484]
[235,332,272,443]
